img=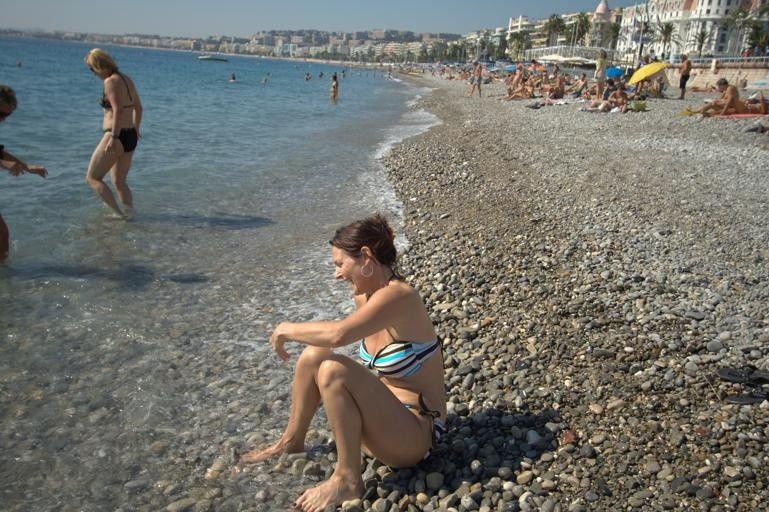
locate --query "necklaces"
[365,274,395,303]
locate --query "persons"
[300,58,406,83]
[228,75,237,82]
[1,83,50,259]
[237,209,450,512]
[329,73,340,104]
[81,48,146,223]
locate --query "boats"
[197,54,227,61]
